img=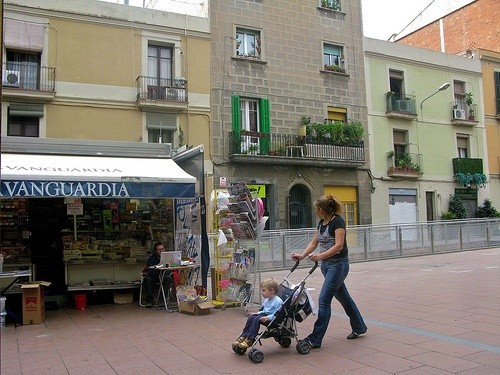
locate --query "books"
[226,180,257,240]
[232,246,255,269]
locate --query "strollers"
[232,255,319,364]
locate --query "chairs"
[138,272,174,307]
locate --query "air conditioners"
[2,70,20,87]
[166,88,185,102]
[453,109,465,119]
[395,100,415,111]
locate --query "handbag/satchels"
[289,292,312,322]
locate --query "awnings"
[1,153,196,198]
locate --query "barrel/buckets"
[73,293,86,310]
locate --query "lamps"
[421,83,451,110]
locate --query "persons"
[291,195,367,350]
[143,242,171,308]
[232,279,283,350]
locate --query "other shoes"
[159,301,165,307]
[146,303,152,308]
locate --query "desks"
[286,146,303,157]
[148,265,200,312]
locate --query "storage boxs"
[15,281,52,325]
[0,297,7,328]
[179,301,214,316]
[211,268,221,300]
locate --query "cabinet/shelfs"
[60,197,175,291]
[0,198,36,293]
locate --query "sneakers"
[347,327,367,339]
[239,338,252,349]
[299,338,321,347]
[232,336,245,346]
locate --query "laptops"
[158,251,182,266]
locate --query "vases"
[270,151,279,156]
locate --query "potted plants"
[466,92,475,120]
[388,151,416,172]
